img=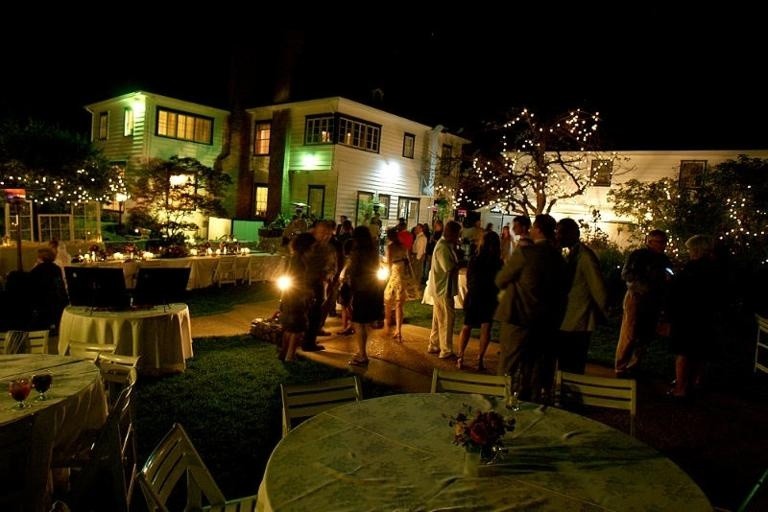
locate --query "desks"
[253,392,714,512]
[422,266,471,310]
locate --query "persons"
[24,248,69,317]
[45,238,61,258]
[273,209,715,402]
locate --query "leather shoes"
[317,330,331,336]
[303,346,325,351]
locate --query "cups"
[506,372,523,412]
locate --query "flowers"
[440,401,517,463]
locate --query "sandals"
[348,359,368,366]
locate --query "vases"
[461,444,482,478]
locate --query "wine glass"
[33,371,53,402]
[10,379,32,412]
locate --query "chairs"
[753,312,768,375]
[430,367,514,400]
[279,374,363,440]
[550,368,637,439]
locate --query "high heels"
[456,356,464,368]
[476,356,483,370]
[394,332,401,342]
